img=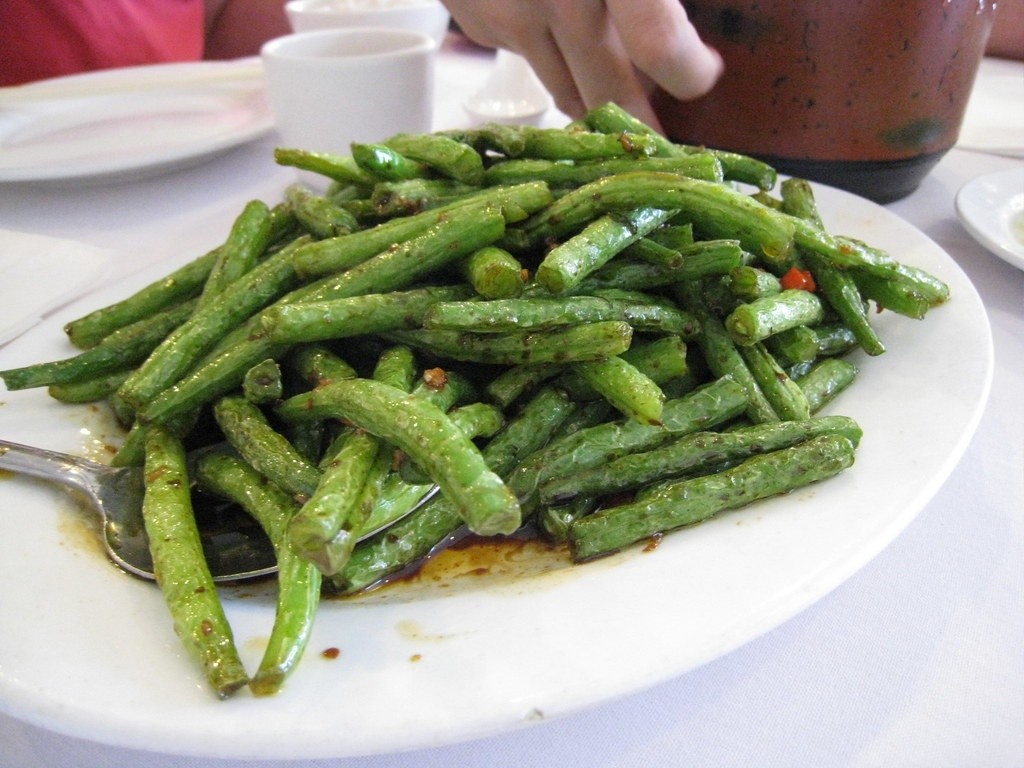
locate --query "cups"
[278,1,453,50]
[261,29,438,193]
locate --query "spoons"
[0,438,444,583]
[468,37,550,127]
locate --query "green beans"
[0,101,950,696]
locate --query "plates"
[953,165,1024,270]
[0,59,283,184]
[0,170,995,764]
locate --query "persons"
[0,0,297,89]
[435,0,725,139]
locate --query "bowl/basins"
[647,0,998,205]
